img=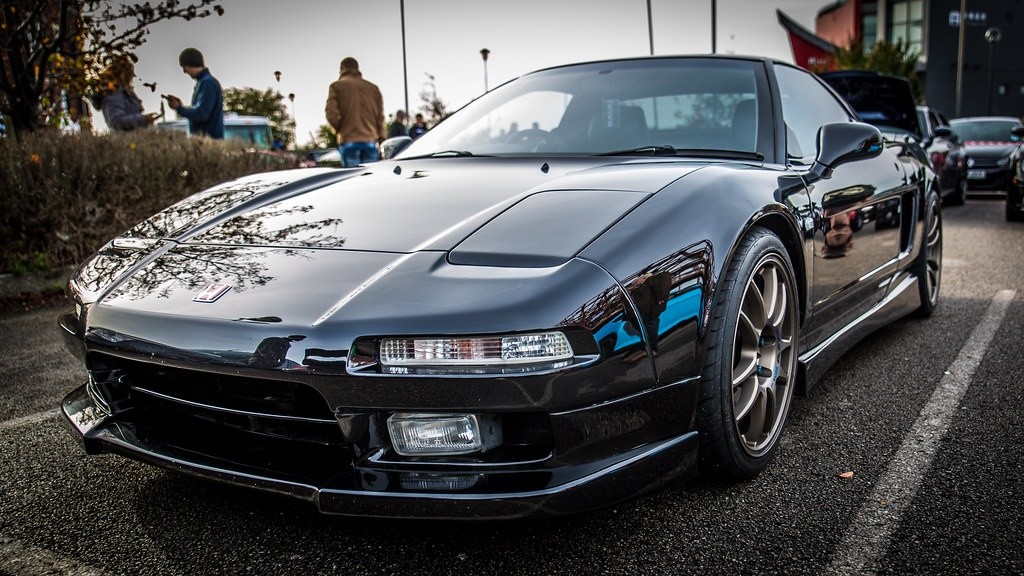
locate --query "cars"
[57,52,942,523]
[820,71,1024,224]
[302,147,345,168]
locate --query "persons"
[325,57,383,168]
[167,47,224,139]
[388,110,407,138]
[409,113,427,140]
[89,61,156,132]
[821,212,855,252]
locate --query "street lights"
[274,69,298,150]
[480,46,492,95]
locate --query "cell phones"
[161,94,180,102]
[153,110,165,119]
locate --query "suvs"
[156,113,286,153]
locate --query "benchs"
[611,105,730,150]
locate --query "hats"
[179,48,203,67]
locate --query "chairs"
[731,99,757,152]
